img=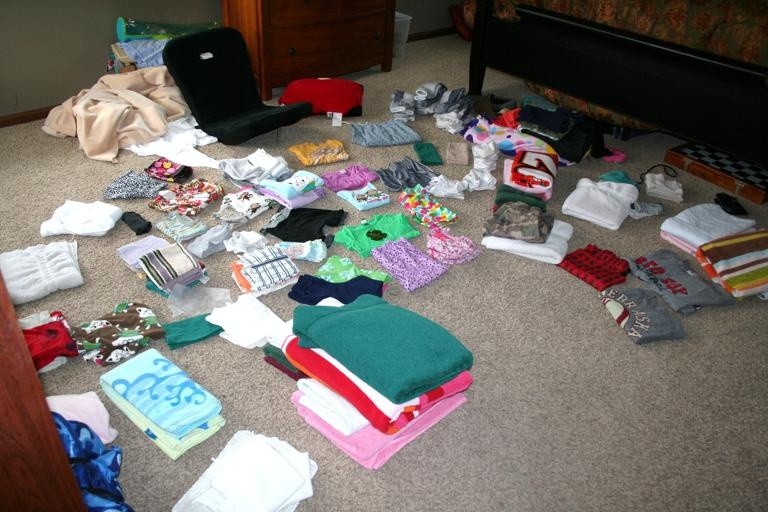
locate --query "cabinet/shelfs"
[223,1,397,101]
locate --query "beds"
[463,0,767,176]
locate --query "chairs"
[162,29,314,146]
